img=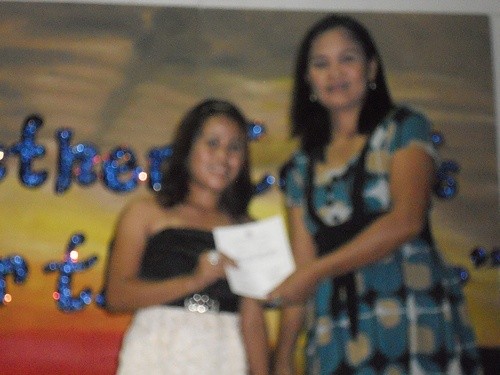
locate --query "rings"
[207,250,219,265]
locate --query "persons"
[267,12,488,375]
[102,95,272,375]
